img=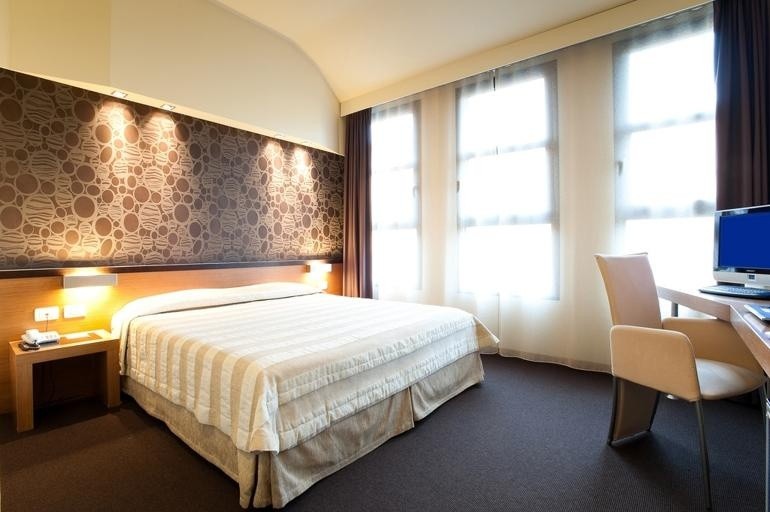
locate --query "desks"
[7,329,121,433]
[650,278,770,374]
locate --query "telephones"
[18,328,60,351]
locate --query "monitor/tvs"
[713,205,770,290]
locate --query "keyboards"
[699,284,770,299]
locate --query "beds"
[111,282,486,509]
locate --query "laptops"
[743,303,770,320]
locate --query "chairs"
[595,253,770,511]
